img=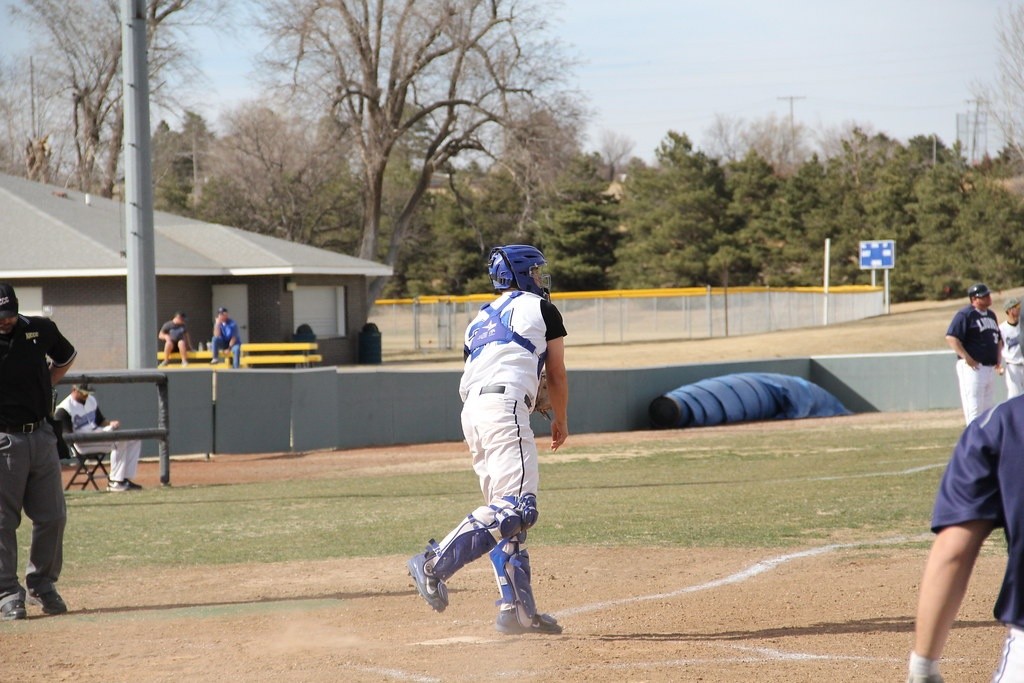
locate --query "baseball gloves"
[533,369,553,423]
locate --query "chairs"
[64,439,110,490]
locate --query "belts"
[0,416,47,435]
[480,386,532,408]
[981,360,998,367]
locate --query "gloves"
[906,652,942,683]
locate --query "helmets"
[488,243,551,299]
[967,284,990,298]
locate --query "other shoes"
[211,358,218,364]
[159,361,167,366]
[181,360,188,366]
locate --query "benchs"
[151,342,323,372]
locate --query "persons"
[945,284,1024,427]
[0,282,76,620]
[408,244,568,635]
[158,311,192,366]
[996,298,1024,399]
[907,296,1024,683]
[55,375,143,492]
[210,307,241,369]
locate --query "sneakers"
[2,600,26,619]
[404,552,445,612]
[497,608,561,635]
[26,584,67,613]
[107,478,142,491]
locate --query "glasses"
[1015,302,1021,309]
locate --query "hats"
[217,307,228,314]
[73,381,97,395]
[0,283,19,318]
[1004,296,1022,312]
